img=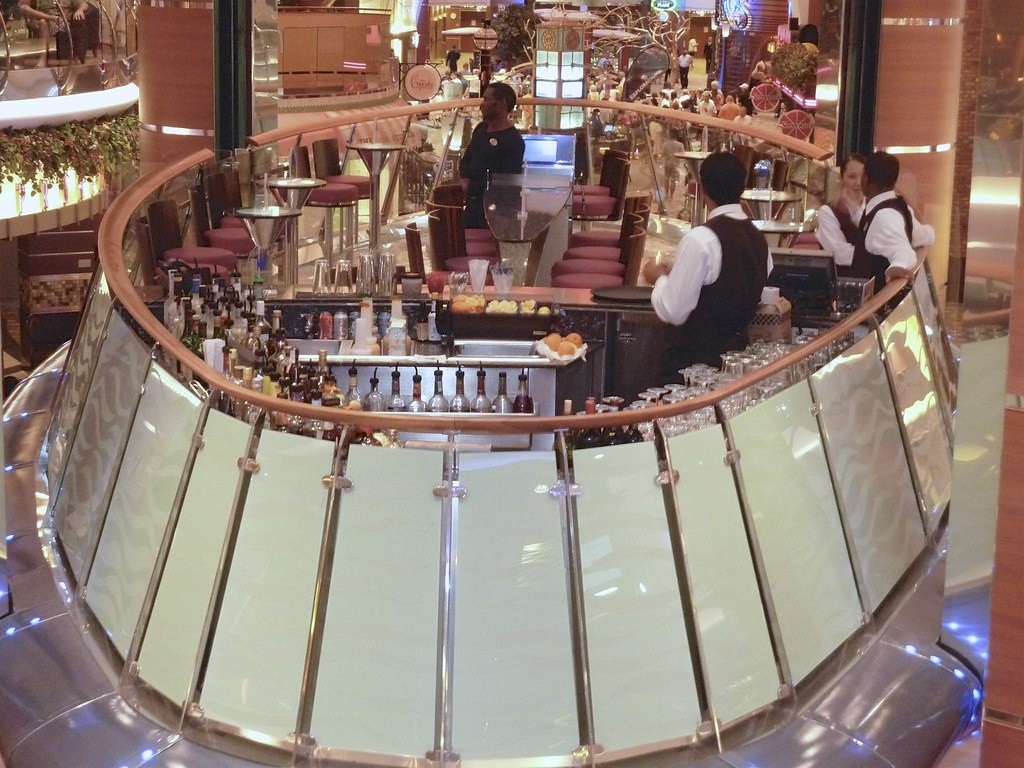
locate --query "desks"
[256,178,328,283]
[484,135,576,285]
[346,143,404,256]
[739,190,801,225]
[752,219,818,247]
[234,207,304,290]
[674,152,711,228]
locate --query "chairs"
[134,136,790,293]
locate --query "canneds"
[320,310,360,340]
[373,311,391,338]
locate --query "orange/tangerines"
[545,332,582,357]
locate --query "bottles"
[163,257,533,446]
[556,396,644,470]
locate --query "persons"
[587,33,772,147]
[458,82,526,256]
[815,150,936,309]
[442,63,532,95]
[446,45,461,74]
[18,0,88,60]
[679,49,694,89]
[642,151,774,385]
[662,129,684,200]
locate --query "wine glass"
[630,330,854,442]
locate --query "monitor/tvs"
[767,247,841,310]
[520,133,577,181]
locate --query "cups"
[377,254,395,297]
[356,255,374,298]
[489,264,514,292]
[334,259,353,296]
[468,259,489,292]
[312,259,332,297]
